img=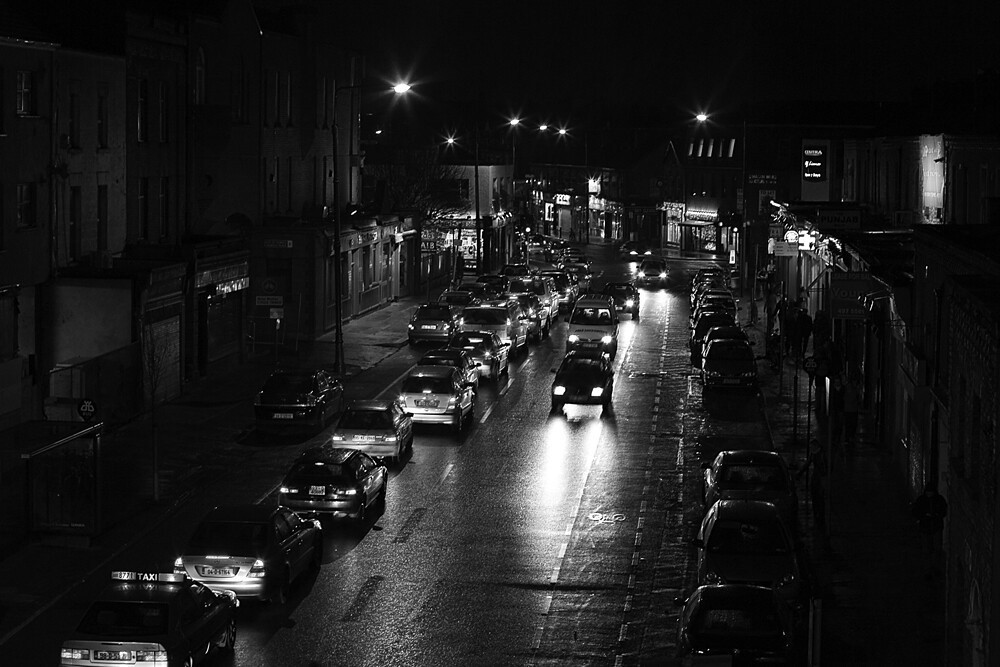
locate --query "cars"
[598,281,640,318]
[416,229,593,395]
[280,447,388,525]
[548,349,615,411]
[673,584,801,666]
[699,448,799,528]
[635,255,671,288]
[408,303,456,346]
[683,256,775,450]
[686,498,804,601]
[60,570,240,667]
[620,242,650,261]
[330,400,414,464]
[172,501,324,609]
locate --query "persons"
[762,286,864,449]
[793,438,828,532]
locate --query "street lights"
[319,206,358,369]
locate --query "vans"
[563,293,619,360]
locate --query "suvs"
[397,366,474,434]
[251,369,344,438]
